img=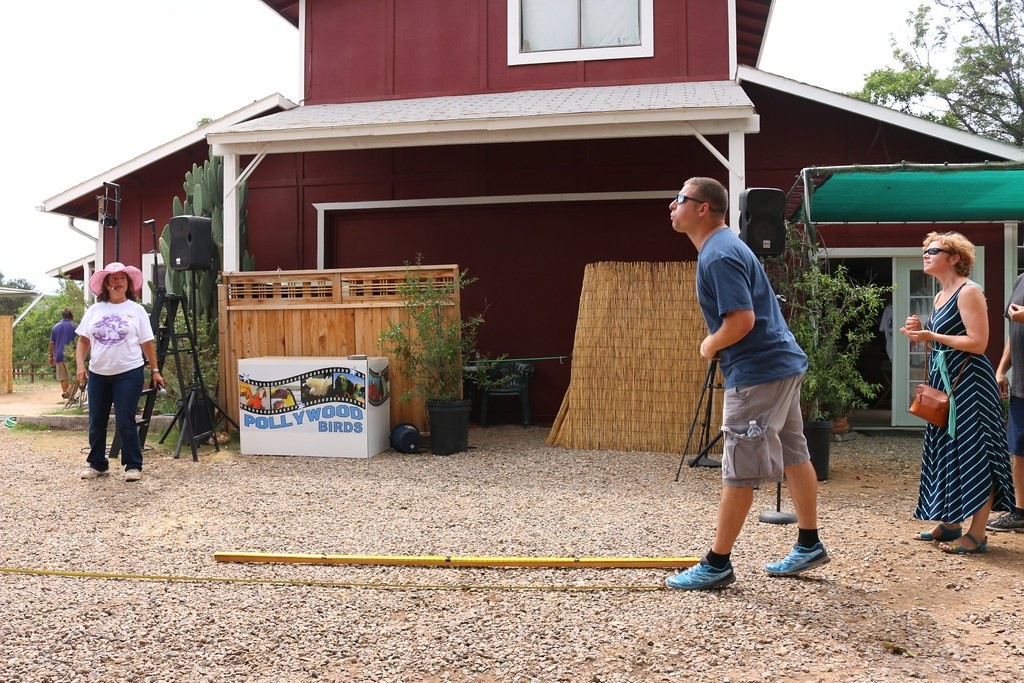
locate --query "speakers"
[738,187,787,258]
[166,215,212,272]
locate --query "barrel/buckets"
[389,423,421,453]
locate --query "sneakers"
[762,539,832,578]
[665,557,736,590]
[125,468,142,481]
[81,468,110,479]
[986,510,1024,533]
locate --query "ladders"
[108,286,221,462]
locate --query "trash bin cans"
[390,423,422,452]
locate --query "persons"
[665,176,831,591]
[48,306,79,404]
[985,270,1024,533]
[75,262,165,482]
[900,231,1016,552]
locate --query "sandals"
[912,525,961,540]
[938,533,988,554]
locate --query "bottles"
[748,420,760,437]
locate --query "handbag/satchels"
[908,383,951,430]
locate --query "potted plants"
[759,217,897,482]
[375,252,520,455]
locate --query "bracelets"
[151,368,160,373]
[49,355,53,357]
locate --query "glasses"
[924,248,952,257]
[675,194,714,214]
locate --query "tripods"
[159,270,240,459]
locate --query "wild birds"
[302,376,332,398]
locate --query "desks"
[237,355,392,460]
[460,364,483,425]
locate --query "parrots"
[335,375,349,398]
[240,386,266,410]
[351,383,362,400]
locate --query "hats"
[89,262,143,298]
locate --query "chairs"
[868,339,892,407]
[482,363,537,428]
